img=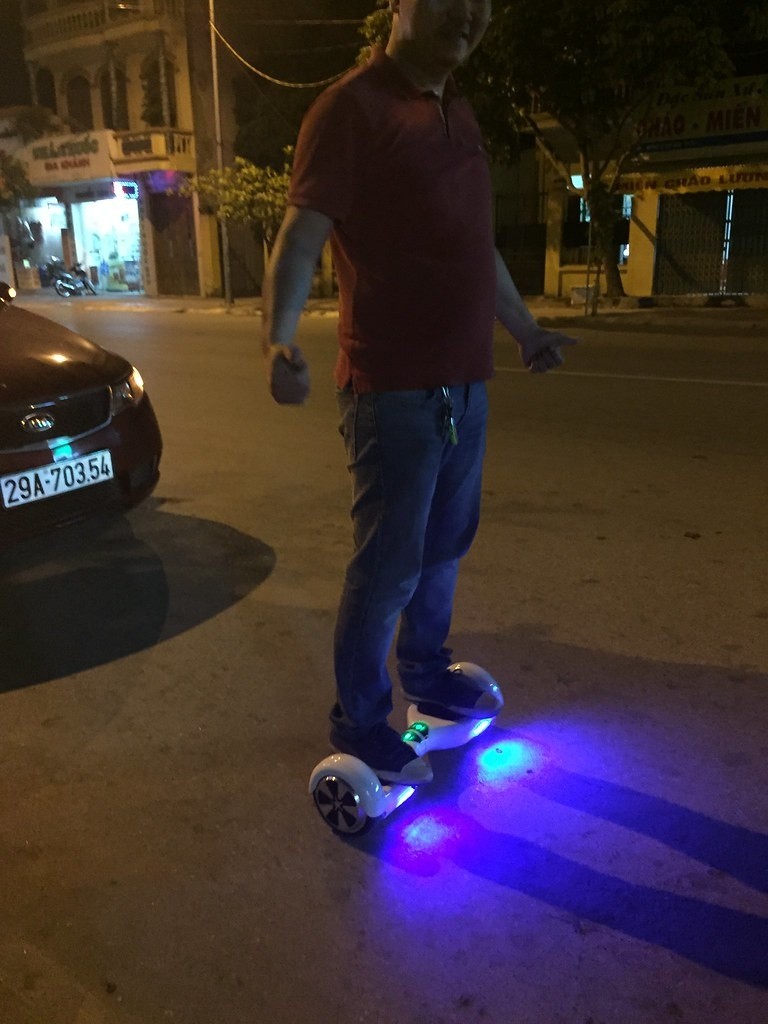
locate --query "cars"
[0,280,164,553]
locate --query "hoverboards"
[307,663,499,837]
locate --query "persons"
[262,0,578,786]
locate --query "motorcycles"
[54,262,100,299]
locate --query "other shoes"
[327,727,434,786]
[400,667,502,718]
